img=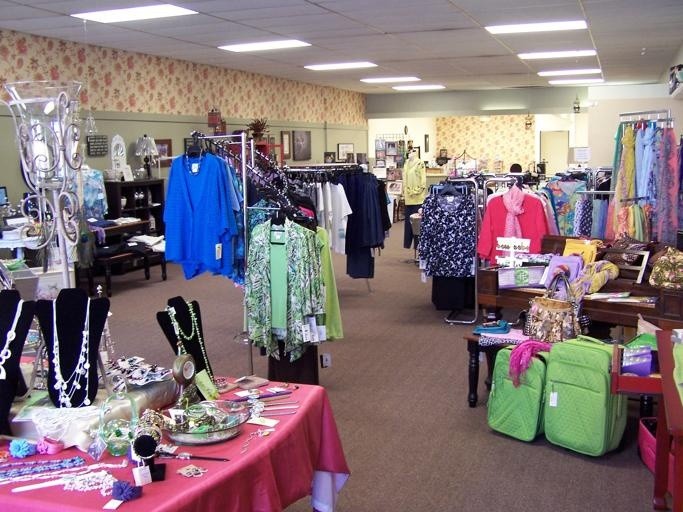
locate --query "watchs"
[231,390,301,418]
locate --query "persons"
[402,153,426,249]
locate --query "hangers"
[428,173,544,199]
[191,126,364,229]
[617,109,674,132]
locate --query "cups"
[120,198,127,209]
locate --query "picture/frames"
[153,138,173,168]
[373,128,427,203]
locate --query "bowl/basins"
[162,399,252,444]
[134,170,147,179]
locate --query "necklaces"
[165,304,211,380]
[51,297,93,409]
[3,446,127,497]
[0,299,27,385]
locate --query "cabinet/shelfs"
[100,175,168,281]
[463,234,683,424]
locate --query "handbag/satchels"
[602,235,651,271]
[524,296,582,343]
[649,246,683,294]
[497,238,597,290]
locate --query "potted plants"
[244,118,267,142]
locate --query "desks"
[0,351,354,511]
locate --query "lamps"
[134,134,157,179]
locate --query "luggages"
[487,335,628,457]
